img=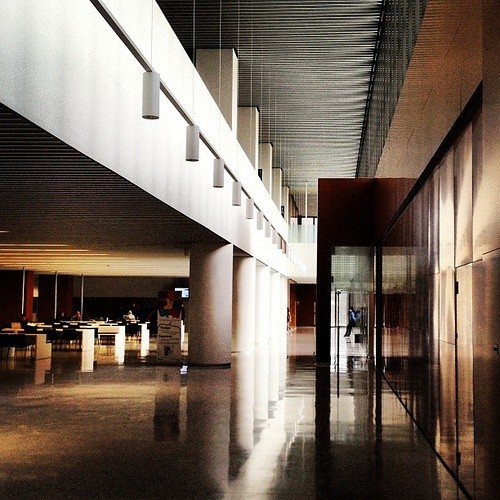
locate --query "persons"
[344,307,356,336]
[125,310,136,321]
[71,311,82,321]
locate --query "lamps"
[139,0,307,277]
[32,272,40,298]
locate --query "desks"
[25,327,95,352]
[98,323,126,347]
[0,331,47,360]
[119,321,151,341]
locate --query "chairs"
[11,321,21,329]
